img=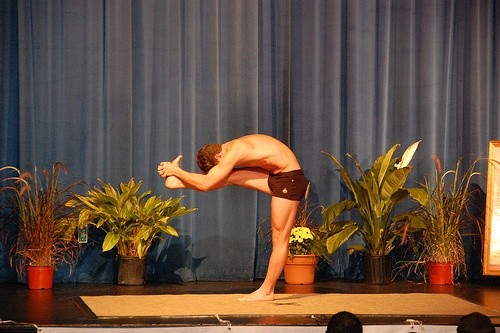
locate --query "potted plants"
[320,139,422,285]
[0,162,89,289]
[56,177,198,286]
[412,153,500,285]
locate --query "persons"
[326,311,363,333]
[456,312,496,333]
[157,134,306,301]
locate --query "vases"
[284,254,320,285]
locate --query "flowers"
[287,225,316,254]
